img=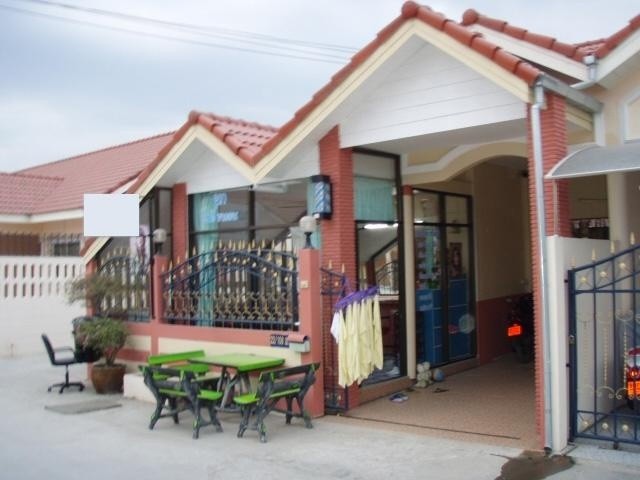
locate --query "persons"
[133,227,148,257]
[446,247,462,279]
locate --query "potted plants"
[65,269,132,394]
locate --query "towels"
[329,291,385,388]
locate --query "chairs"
[40,333,84,394]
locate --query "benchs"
[137,350,321,443]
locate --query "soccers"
[432,368,445,382]
